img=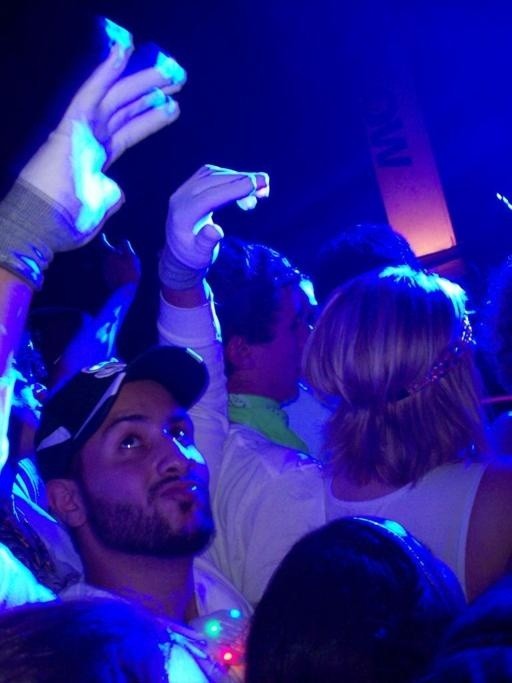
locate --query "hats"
[34,345,209,478]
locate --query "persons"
[0,40,510,683]
